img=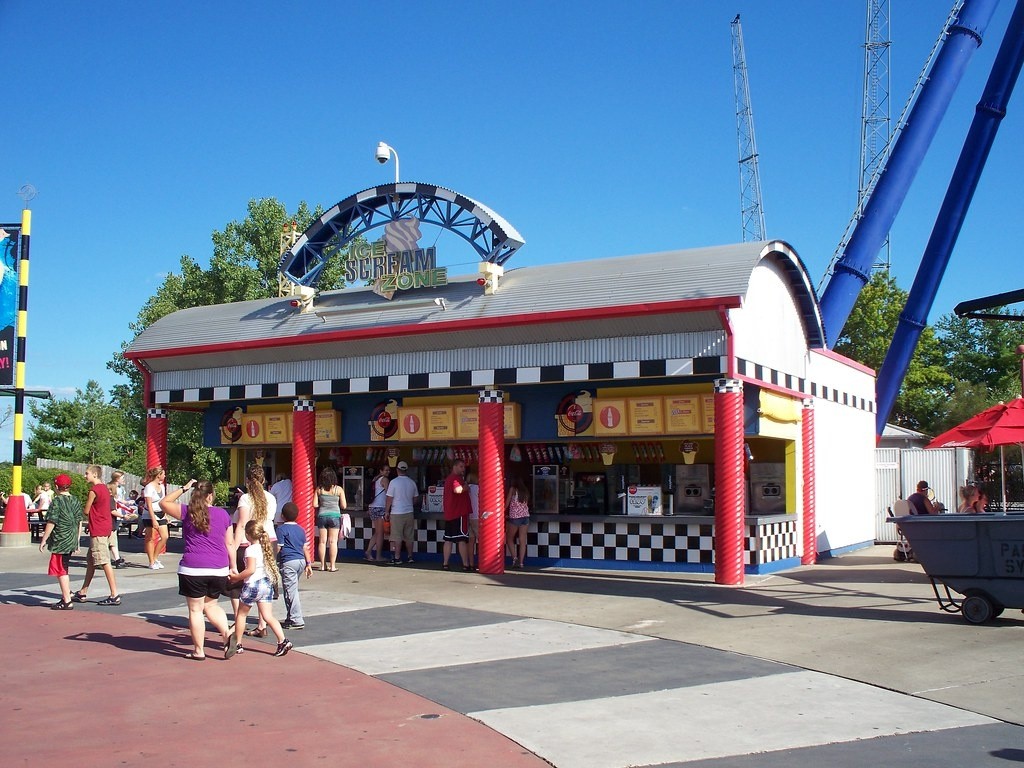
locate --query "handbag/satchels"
[155,511,165,519]
[384,521,390,535]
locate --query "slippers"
[374,556,387,562]
[183,652,205,660]
[363,557,373,561]
[223,633,237,659]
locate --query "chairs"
[894,500,909,517]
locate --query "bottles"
[647,495,658,513]
[251,421,255,436]
[409,415,415,432]
[607,407,613,427]
[524,443,601,464]
[631,441,665,462]
[413,447,478,466]
[366,447,383,465]
[422,495,425,509]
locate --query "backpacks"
[365,475,384,504]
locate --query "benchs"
[119,521,184,539]
[27,520,48,540]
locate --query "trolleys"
[884,510,1024,626]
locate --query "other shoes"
[155,560,165,569]
[148,564,158,570]
[136,535,145,539]
[131,531,138,538]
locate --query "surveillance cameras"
[375,146,390,163]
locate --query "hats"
[917,481,932,491]
[55,474,72,488]
[397,462,408,470]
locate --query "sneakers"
[280,621,304,629]
[274,638,293,656]
[236,644,243,654]
[407,557,415,563]
[386,556,403,564]
[116,559,132,569]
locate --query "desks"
[25,509,49,531]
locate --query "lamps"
[477,278,492,287]
[314,310,327,323]
[434,297,446,311]
[283,221,297,233]
[290,295,316,309]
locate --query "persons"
[441,458,478,571]
[365,461,419,564]
[505,476,531,569]
[314,468,347,572]
[908,481,941,515]
[0,482,55,519]
[75,465,122,606]
[39,474,83,610]
[958,485,986,514]
[160,464,313,661]
[96,465,171,569]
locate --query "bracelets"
[306,561,311,565]
[181,486,186,492]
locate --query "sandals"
[98,595,121,605]
[46,596,73,610]
[511,557,518,569]
[470,566,475,571]
[517,564,524,568]
[442,563,448,570]
[462,566,469,572]
[71,591,87,604]
[246,627,268,638]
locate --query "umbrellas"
[923,395,1024,516]
